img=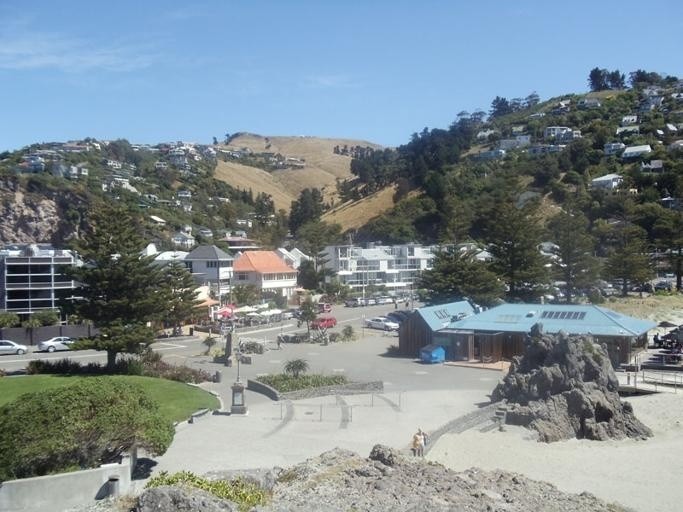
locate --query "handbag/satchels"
[424,435,430,445]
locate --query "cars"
[315,303,332,313]
[345,294,420,307]
[311,316,337,329]
[38,336,74,353]
[523,278,673,301]
[281,307,302,320]
[365,310,411,331]
[0,340,27,355]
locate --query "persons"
[654,333,662,347]
[410,426,428,457]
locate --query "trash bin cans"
[216,370,222,383]
[108,475,120,497]
[190,328,193,336]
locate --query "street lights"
[234,349,242,386]
